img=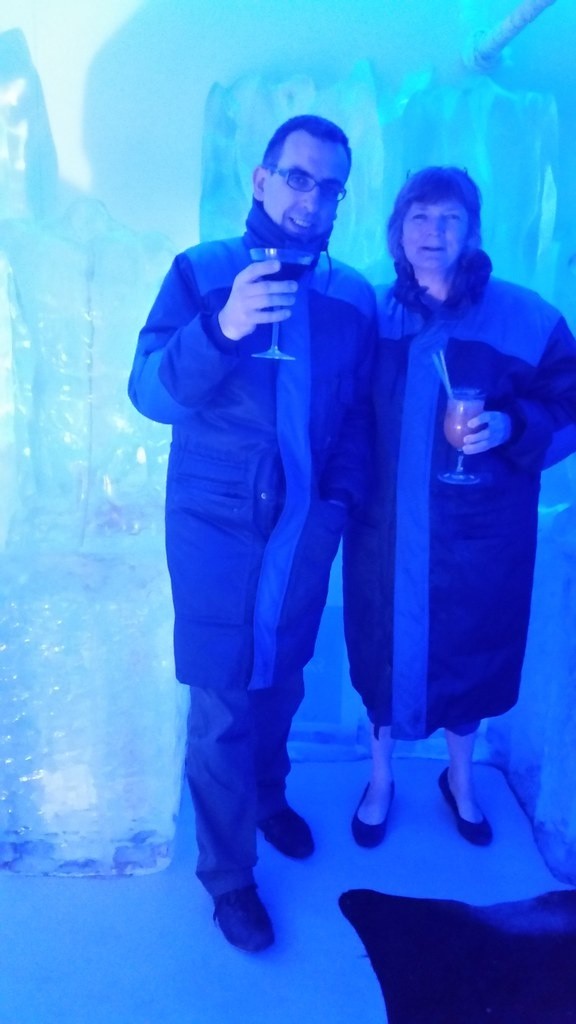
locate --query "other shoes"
[256,804,322,860]
[440,768,493,846]
[351,783,395,849]
[211,883,276,951]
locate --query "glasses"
[271,167,350,203]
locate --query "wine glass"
[250,248,315,360]
[438,388,486,485]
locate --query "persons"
[127,113,381,955]
[346,165,573,846]
